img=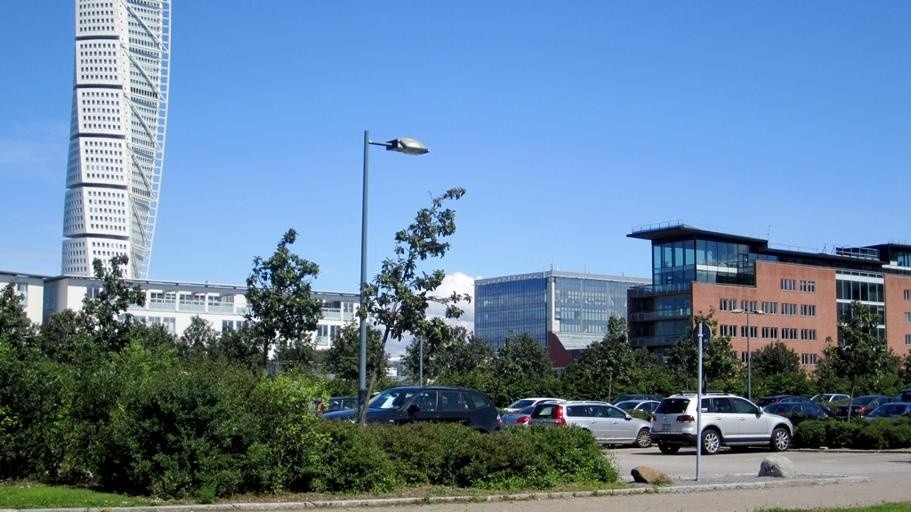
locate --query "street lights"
[356,130,431,428]
[731,307,766,403]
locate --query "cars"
[322,397,359,413]
[324,387,498,435]
